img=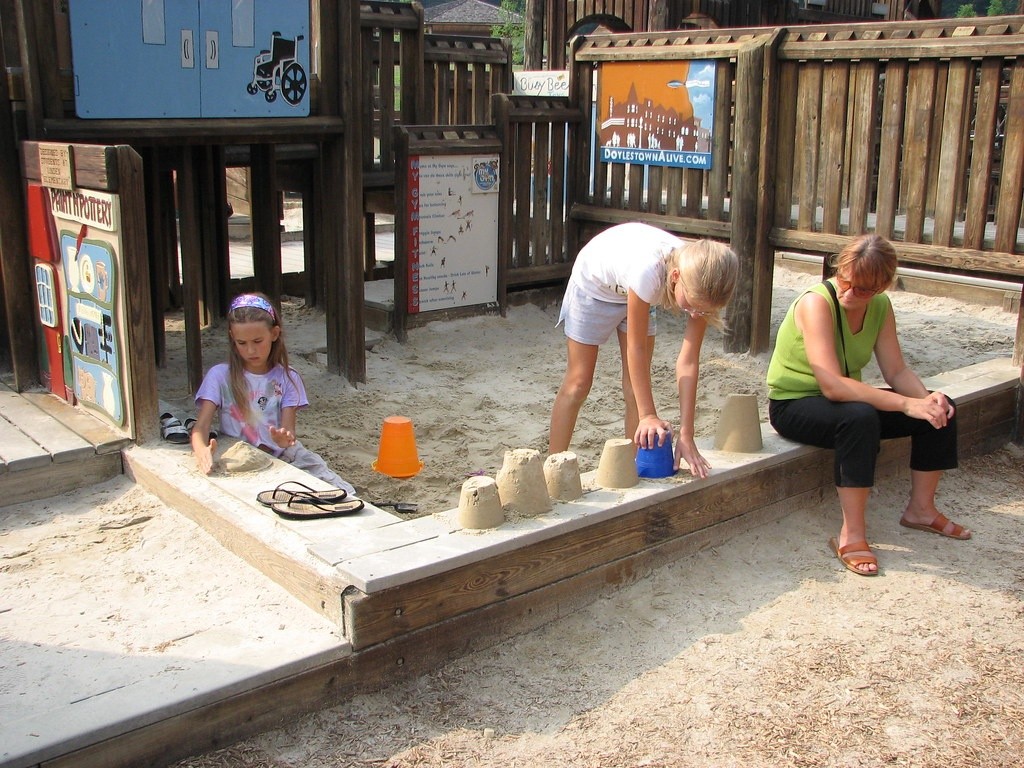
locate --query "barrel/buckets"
[373,416,421,478]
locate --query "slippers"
[828,536,878,576]
[255,480,363,521]
[899,511,971,540]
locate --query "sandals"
[161,412,219,443]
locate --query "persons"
[765,234,971,576]
[190,293,358,498]
[548,223,738,481]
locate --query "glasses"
[837,276,883,299]
[682,284,719,316]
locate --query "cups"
[638,435,675,478]
